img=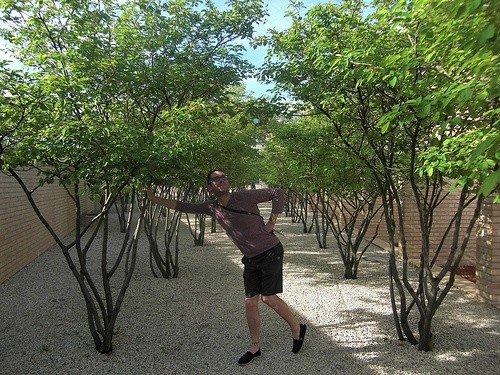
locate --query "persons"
[146,168,307,366]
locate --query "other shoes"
[291,323,307,353]
[239,348,261,365]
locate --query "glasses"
[208,175,229,184]
